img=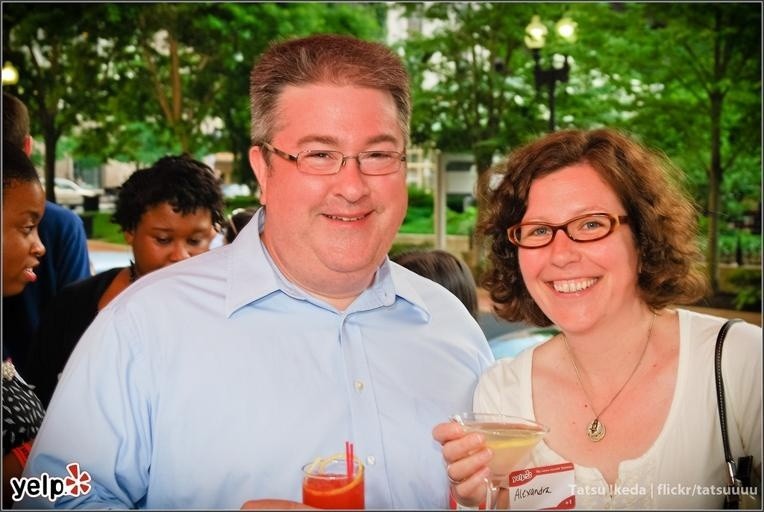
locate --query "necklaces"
[560,309,655,444]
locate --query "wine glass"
[447,410,550,509]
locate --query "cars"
[39,176,107,210]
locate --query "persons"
[16,36,493,510]
[391,250,480,323]
[432,129,763,512]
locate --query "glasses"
[263,141,405,175]
[507,211,630,249]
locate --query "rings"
[445,464,466,485]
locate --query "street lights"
[523,14,577,132]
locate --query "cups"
[303,456,366,511]
